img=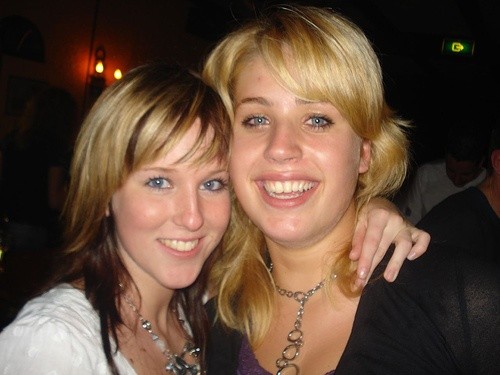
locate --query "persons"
[0,62,431,375]
[0,14,71,321]
[199,3,500,375]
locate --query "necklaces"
[117,281,200,375]
[264,257,339,375]
